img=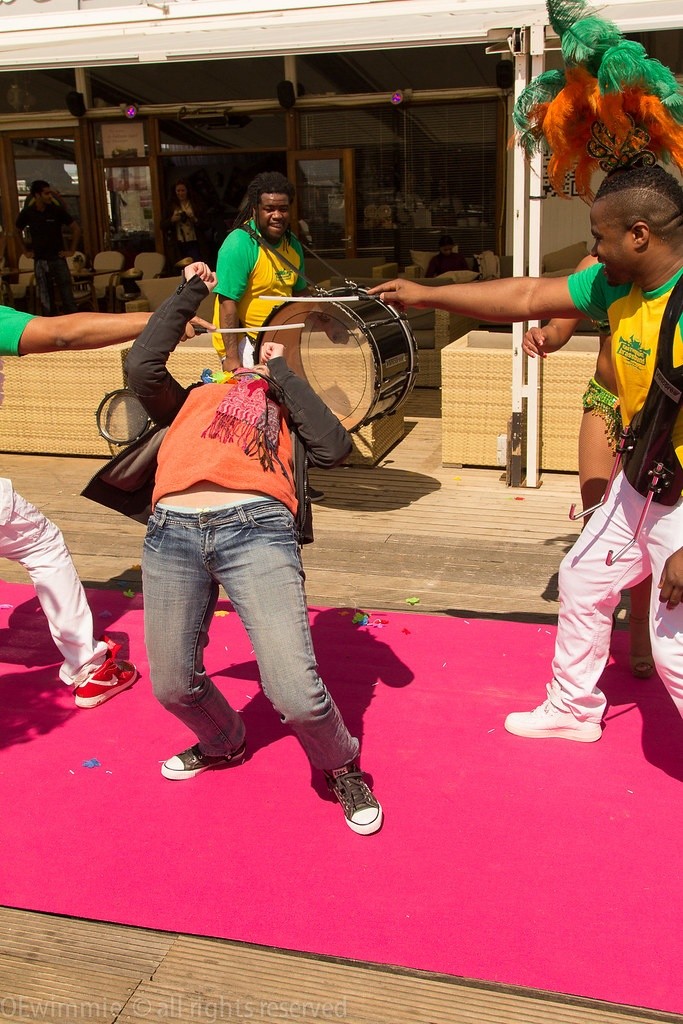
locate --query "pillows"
[436,270,480,283]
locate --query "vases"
[73,261,84,271]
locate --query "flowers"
[73,254,83,262]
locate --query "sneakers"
[75,635,138,708]
[161,740,246,780]
[322,762,383,835]
[505,683,602,743]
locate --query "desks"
[68,268,122,312]
[1,266,34,308]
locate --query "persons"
[0,304,217,709]
[523,254,655,679]
[368,166,683,742]
[211,172,326,501]
[164,179,202,268]
[424,235,470,278]
[80,262,381,835]
[15,180,79,317]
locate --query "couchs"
[125,249,513,387]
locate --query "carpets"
[0,580,683,1015]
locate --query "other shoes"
[306,487,323,503]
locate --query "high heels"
[630,615,655,677]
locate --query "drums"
[251,287,420,434]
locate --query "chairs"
[3,252,165,313]
